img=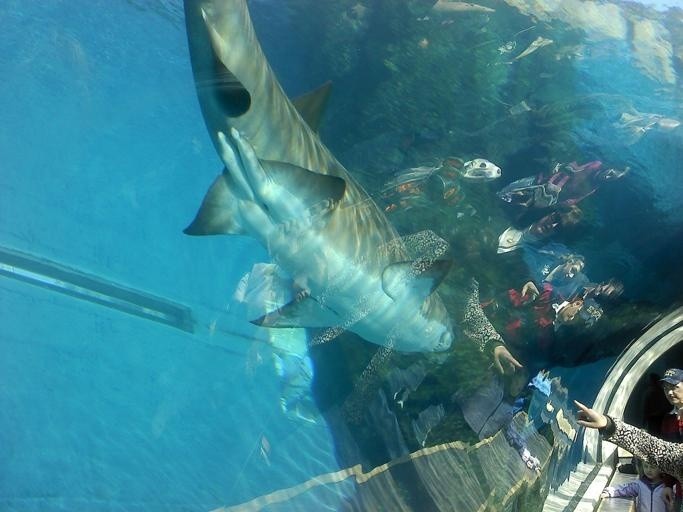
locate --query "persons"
[306,160,634,477]
[573,367,683,511]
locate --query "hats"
[660,369,683,385]
